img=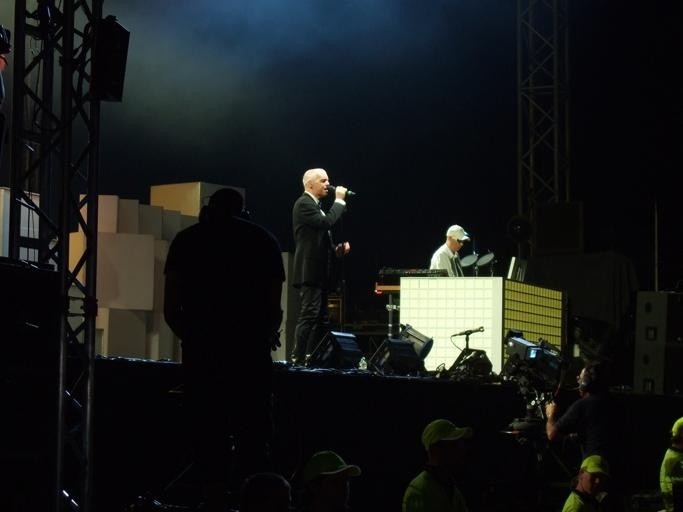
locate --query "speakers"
[367,339,417,376]
[448,348,491,374]
[633,290,683,395]
[307,330,362,370]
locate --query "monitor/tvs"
[507,257,528,282]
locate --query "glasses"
[453,237,463,243]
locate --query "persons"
[162,187,286,364]
[544,364,614,470]
[287,166,351,370]
[235,472,294,511]
[560,454,610,512]
[401,418,474,512]
[429,224,472,277]
[658,416,682,512]
[298,450,363,510]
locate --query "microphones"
[327,185,356,195]
[458,327,484,335]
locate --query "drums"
[461,254,478,267]
[476,253,495,267]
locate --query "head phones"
[581,365,591,391]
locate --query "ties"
[454,258,463,277]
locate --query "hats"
[671,415,683,438]
[302,450,362,478]
[421,418,472,450]
[446,224,470,241]
[579,453,610,476]
